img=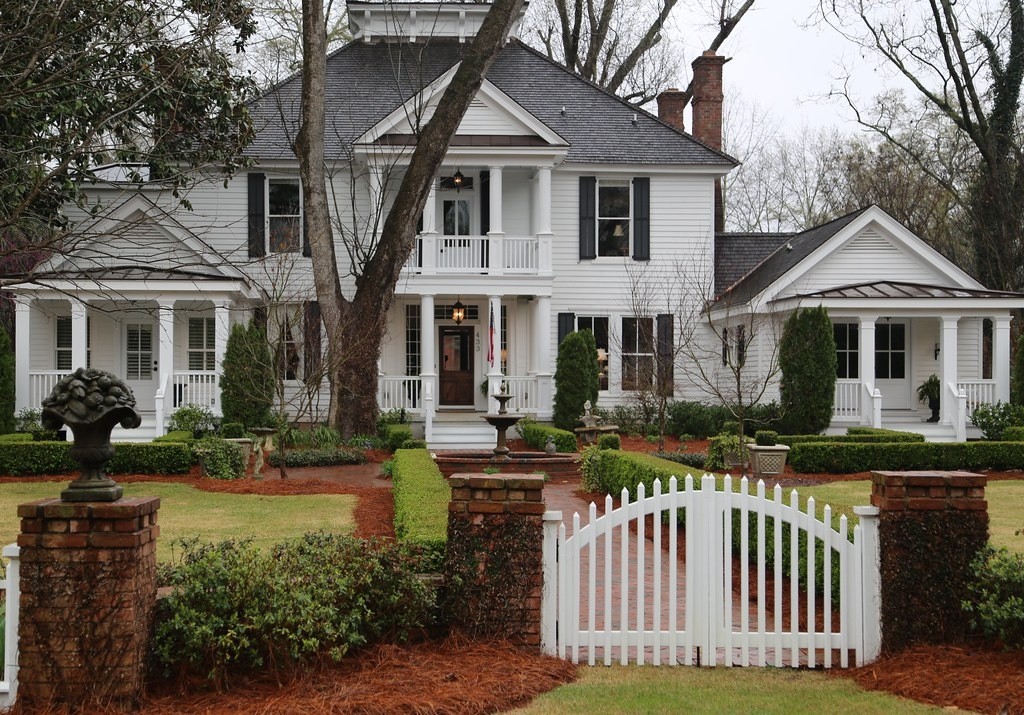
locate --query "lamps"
[452,294,464,327]
[613,225,625,249]
[933,335,940,360]
[596,347,607,376]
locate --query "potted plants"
[713,421,752,466]
[917,373,941,423]
[746,429,790,476]
[453,165,463,194]
[217,423,251,468]
